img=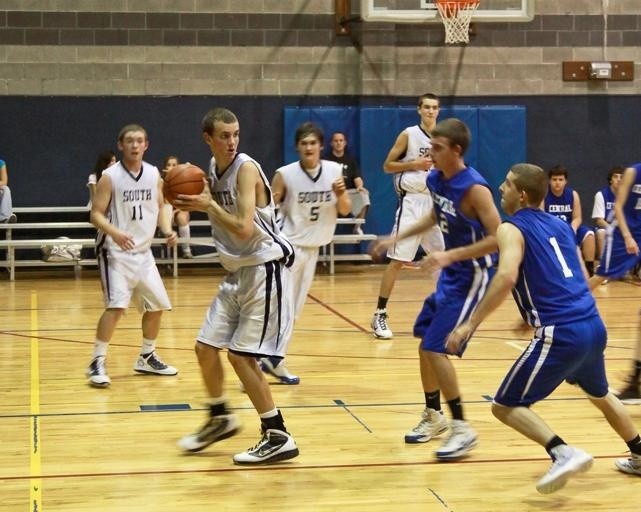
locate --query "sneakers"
[614,450,641,475]
[371,308,394,340]
[234,410,298,465]
[613,361,641,405]
[5,215,17,224]
[177,403,243,452]
[434,420,480,460]
[536,445,594,494]
[85,356,111,387]
[256,356,300,385]
[405,407,448,443]
[134,350,178,376]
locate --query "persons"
[367,94,449,343]
[87,125,179,390]
[162,156,193,257]
[86,150,118,211]
[327,130,372,238]
[539,167,594,284]
[171,105,300,466]
[582,158,641,292]
[613,300,641,401]
[442,162,641,498]
[237,124,352,397]
[368,116,501,462]
[587,169,625,263]
[0,158,17,226]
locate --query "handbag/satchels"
[41,236,83,262]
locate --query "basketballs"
[162,164,206,209]
